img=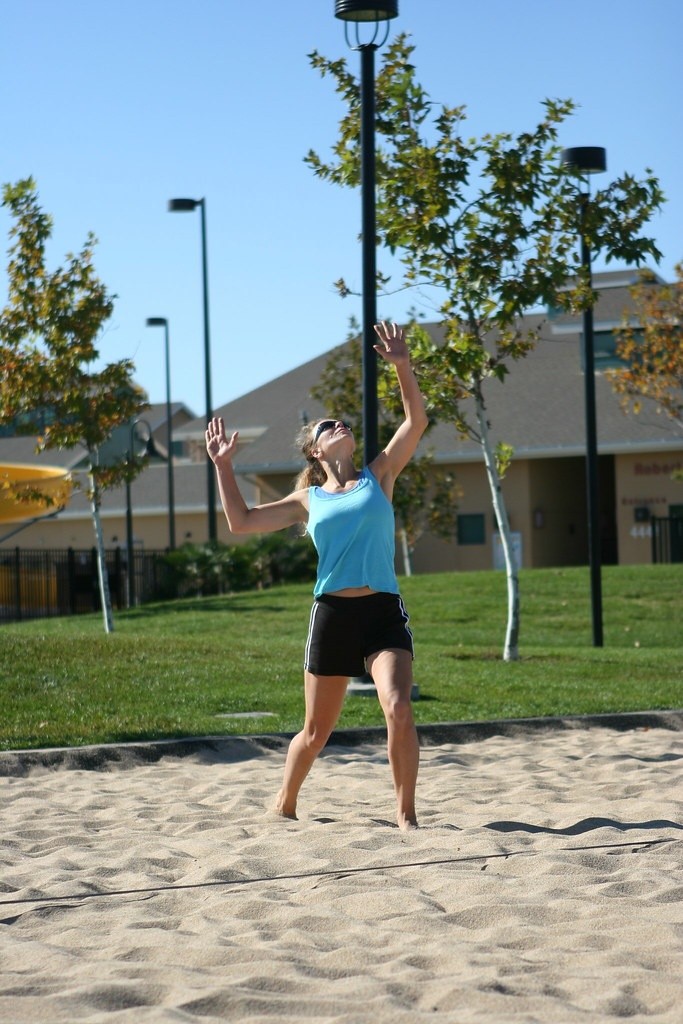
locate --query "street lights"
[556,144,609,646]
[327,0,406,689]
[124,417,162,619]
[146,316,181,602]
[168,194,221,595]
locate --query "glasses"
[314,420,353,442]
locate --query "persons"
[205,321,430,831]
